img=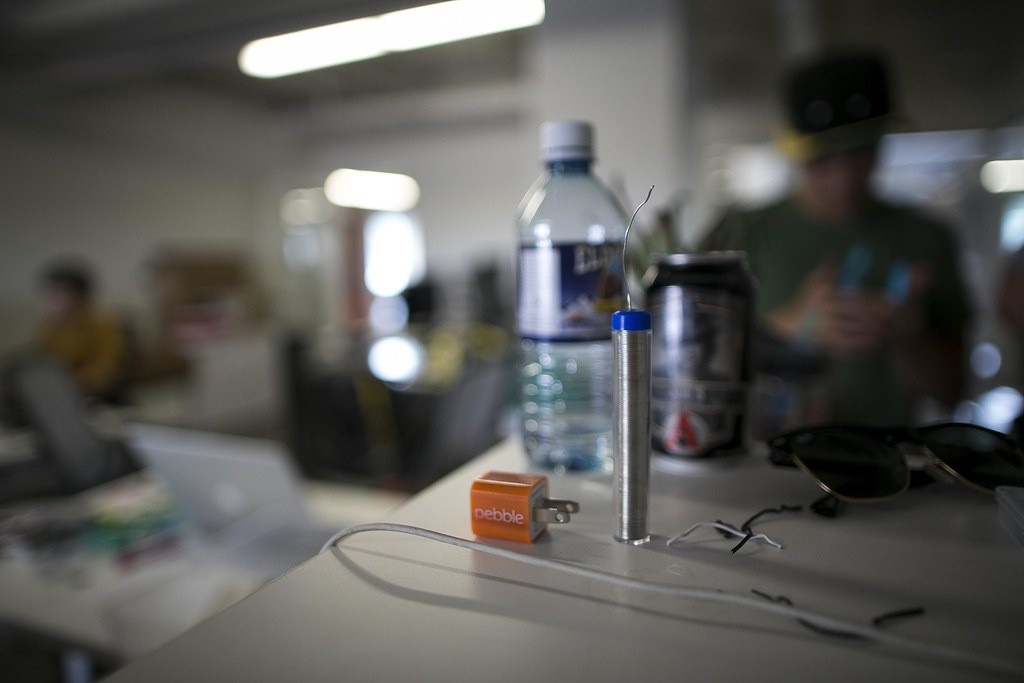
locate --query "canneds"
[639,251,758,474]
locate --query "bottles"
[509,120,631,473]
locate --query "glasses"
[766,423,1024,516]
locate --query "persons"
[19,261,143,412]
[699,42,971,460]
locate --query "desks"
[0,434,410,682]
[97,436,1023,682]
